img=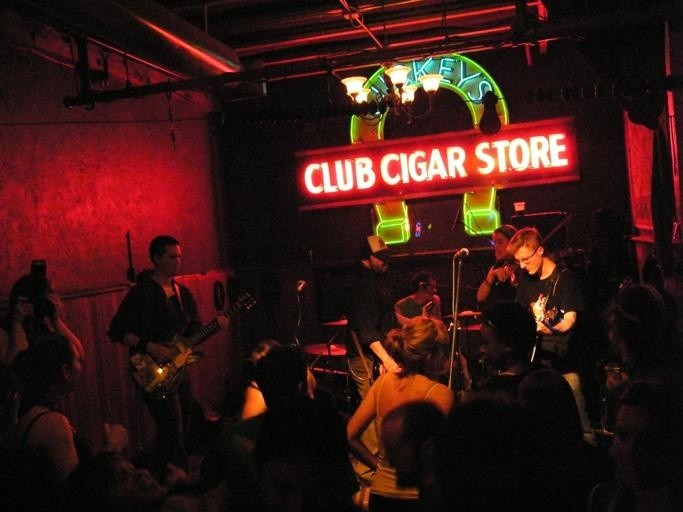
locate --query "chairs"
[446,308,484,358]
[306,317,350,394]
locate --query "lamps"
[340,1,444,127]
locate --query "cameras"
[24,260,53,317]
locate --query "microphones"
[419,282,431,288]
[454,247,469,259]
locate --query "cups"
[604,361,622,382]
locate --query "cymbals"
[440,310,481,319]
[304,343,347,357]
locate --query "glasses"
[515,249,537,265]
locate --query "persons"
[345,233,400,345]
[237,313,317,421]
[103,235,214,438]
[345,316,455,512]
[446,225,683,511]
[377,400,449,511]
[1,270,210,512]
[392,270,443,323]
[232,343,353,512]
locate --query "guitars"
[130,294,255,399]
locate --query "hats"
[360,235,392,260]
